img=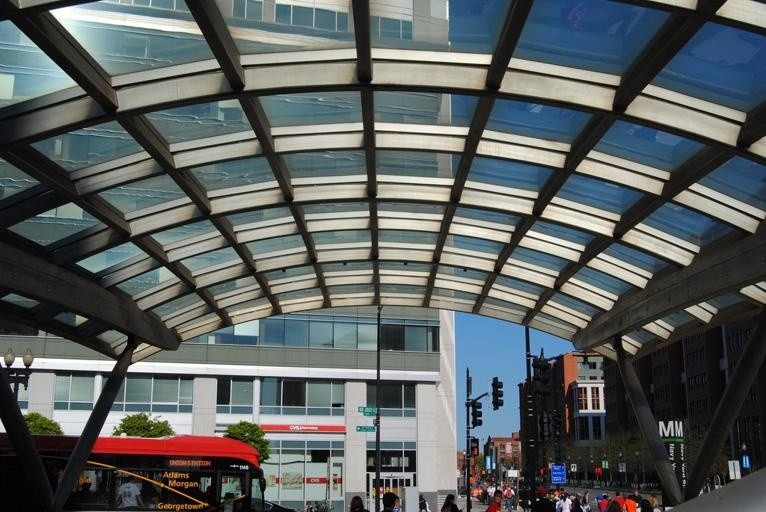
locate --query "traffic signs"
[550,463,567,484]
[357,406,377,432]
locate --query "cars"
[221,495,294,512]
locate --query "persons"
[382,492,400,511]
[440,494,459,511]
[419,495,427,510]
[306,500,324,511]
[350,496,366,510]
[65,471,211,512]
[520,484,590,511]
[596,487,662,511]
[470,473,516,511]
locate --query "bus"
[0,434,265,511]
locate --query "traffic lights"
[493,377,503,410]
[470,438,479,458]
[471,402,482,426]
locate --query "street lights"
[569,452,641,489]
